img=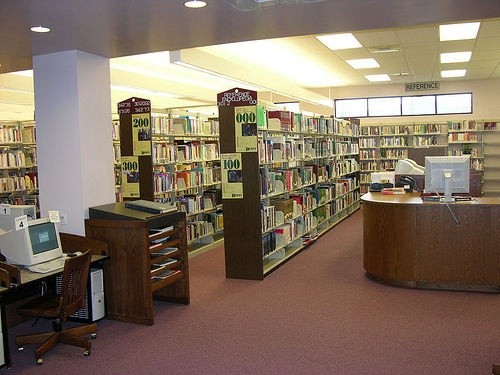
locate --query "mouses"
[73,251,83,256]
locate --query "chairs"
[15,248,99,364]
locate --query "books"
[0,110,497,279]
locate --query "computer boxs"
[39,268,105,323]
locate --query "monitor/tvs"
[0,217,63,266]
[395,160,425,175]
[425,156,471,202]
[406,159,426,170]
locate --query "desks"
[0,232,109,369]
[362,190,500,293]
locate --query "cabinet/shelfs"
[0,88,500,326]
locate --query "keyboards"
[30,257,70,273]
